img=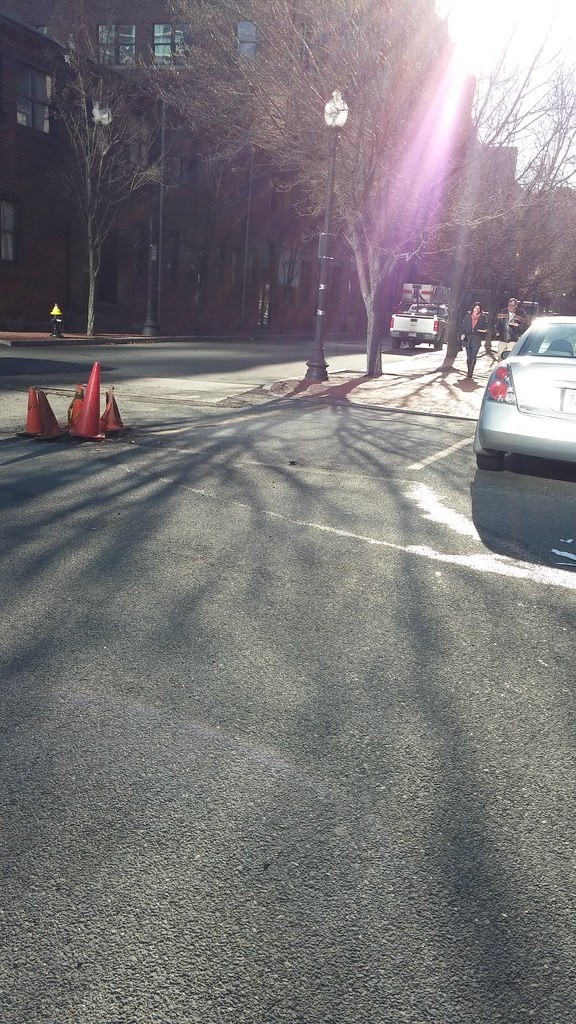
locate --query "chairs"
[547,339,574,356]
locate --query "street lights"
[305,85,350,380]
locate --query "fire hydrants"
[50,302,64,338]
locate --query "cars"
[462,301,562,337]
[473,315,576,472]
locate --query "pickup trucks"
[390,304,450,351]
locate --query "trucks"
[399,282,451,311]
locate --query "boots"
[467,359,475,378]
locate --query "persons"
[496,298,526,364]
[462,302,487,379]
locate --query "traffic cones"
[25,361,124,441]
